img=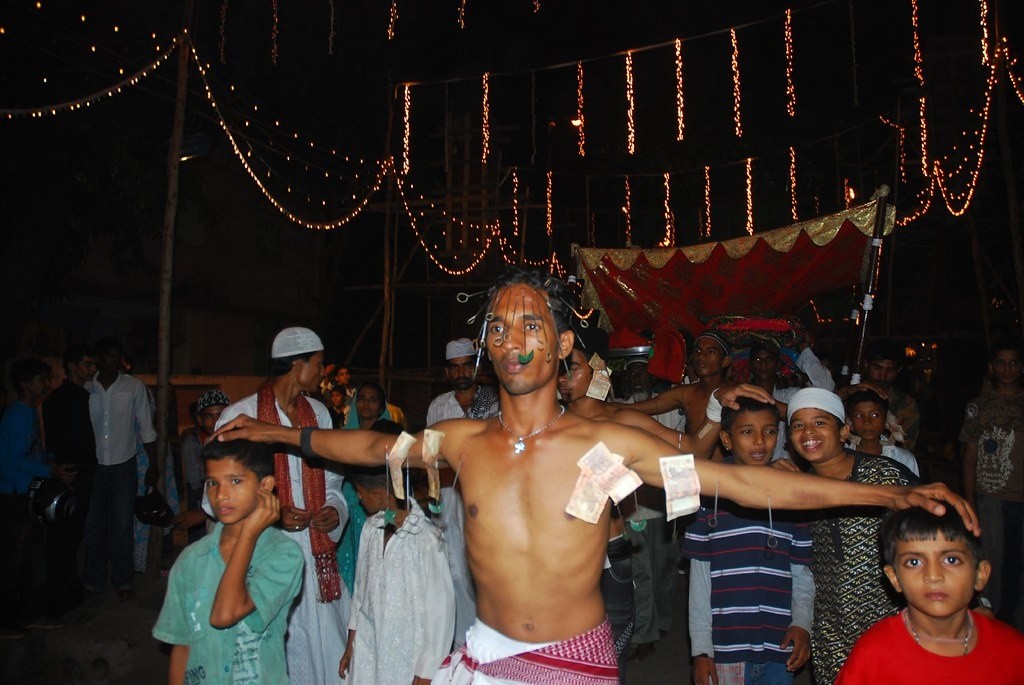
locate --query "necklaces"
[498,404,565,454]
[905,610,970,655]
[902,607,974,643]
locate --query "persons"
[0,266,1024,685]
[201,266,982,685]
[152,437,306,685]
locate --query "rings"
[295,526,299,530]
[294,515,298,520]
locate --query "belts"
[607,547,632,562]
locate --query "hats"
[196,389,231,413]
[623,357,648,370]
[271,326,325,361]
[694,329,733,356]
[786,387,845,425]
[446,338,478,360]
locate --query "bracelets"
[707,387,722,423]
[678,431,682,450]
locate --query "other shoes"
[0,622,27,639]
[85,579,105,593]
[628,642,654,660]
[120,588,138,602]
[25,615,66,629]
